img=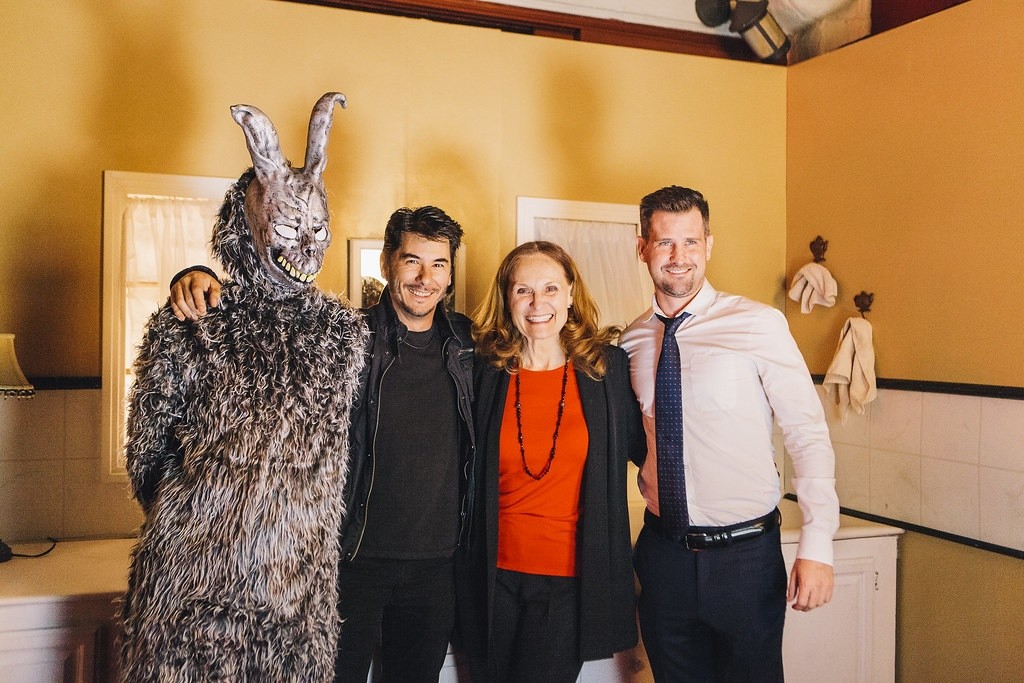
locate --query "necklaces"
[403,321,437,349]
[516,355,568,480]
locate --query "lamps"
[0,333,37,563]
[695,0,791,64]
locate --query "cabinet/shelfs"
[0,603,126,682]
[429,537,896,682]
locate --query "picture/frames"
[349,238,467,315]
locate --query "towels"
[822,316,877,429]
[788,262,838,314]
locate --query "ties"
[654,312,693,543]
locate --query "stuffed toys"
[111,169,369,683]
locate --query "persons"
[615,186,840,683]
[168,206,484,683]
[449,241,647,683]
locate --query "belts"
[644,507,779,550]
